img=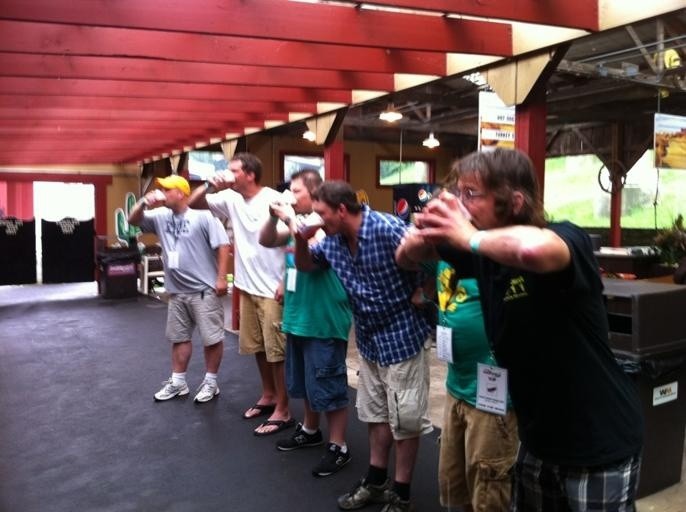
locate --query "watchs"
[268,212,279,223]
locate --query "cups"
[408,191,473,243]
[293,210,324,234]
[276,188,297,207]
[203,170,233,190]
[142,187,167,207]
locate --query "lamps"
[377,100,402,124]
[300,130,314,143]
[419,131,441,151]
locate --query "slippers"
[243,402,276,419]
[253,415,295,437]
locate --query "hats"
[156,174,190,197]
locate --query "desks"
[596,249,662,282]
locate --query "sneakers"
[311,442,352,477]
[193,382,220,403]
[337,476,389,510]
[154,378,190,401]
[382,490,416,512]
[276,425,323,451]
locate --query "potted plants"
[652,213,686,285]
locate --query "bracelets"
[136,195,147,209]
[204,178,217,193]
[216,273,229,280]
[282,216,294,225]
[470,230,487,255]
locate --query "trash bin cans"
[599,278,686,502]
[97,236,140,299]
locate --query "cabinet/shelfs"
[136,254,165,295]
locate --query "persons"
[392,148,645,510]
[288,179,440,510]
[124,173,233,406]
[392,156,522,510]
[185,154,297,437]
[256,166,357,479]
